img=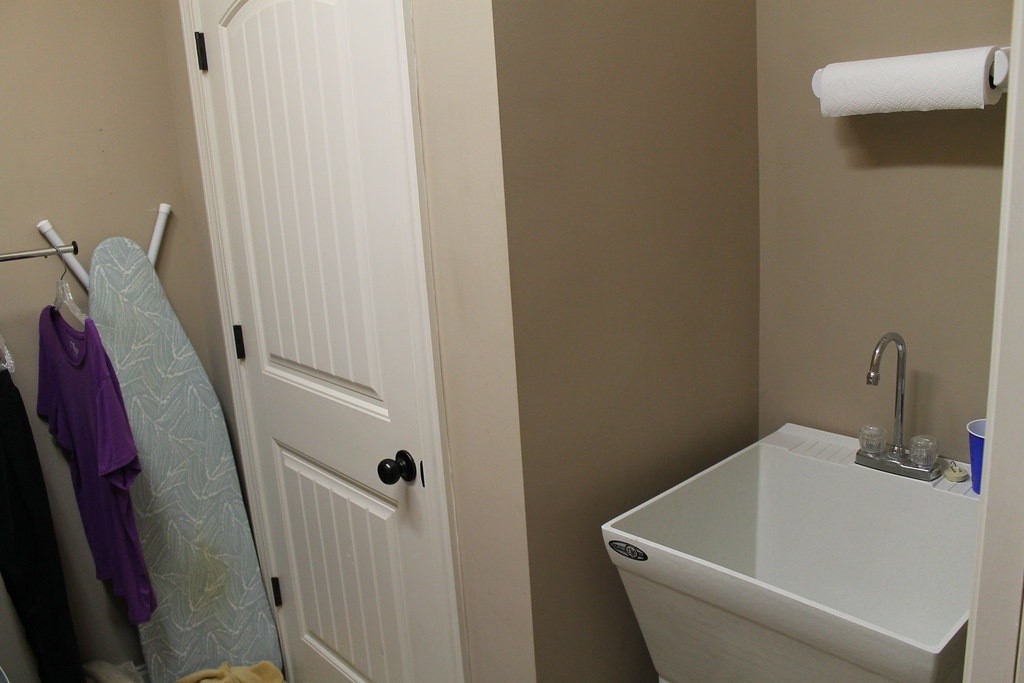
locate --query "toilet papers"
[819,42,1003,120]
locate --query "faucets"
[865,331,908,476]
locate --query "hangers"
[0,334,16,373]
[44,246,88,327]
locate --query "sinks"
[600,420,981,654]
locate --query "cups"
[966,418,987,495]
